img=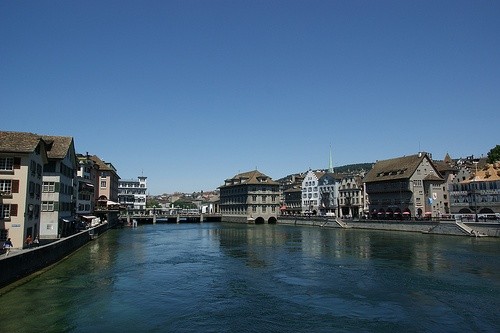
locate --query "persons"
[24,235,32,250]
[3,238,13,257]
[59,228,62,238]
[33,234,40,247]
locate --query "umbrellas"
[425,211,432,221]
